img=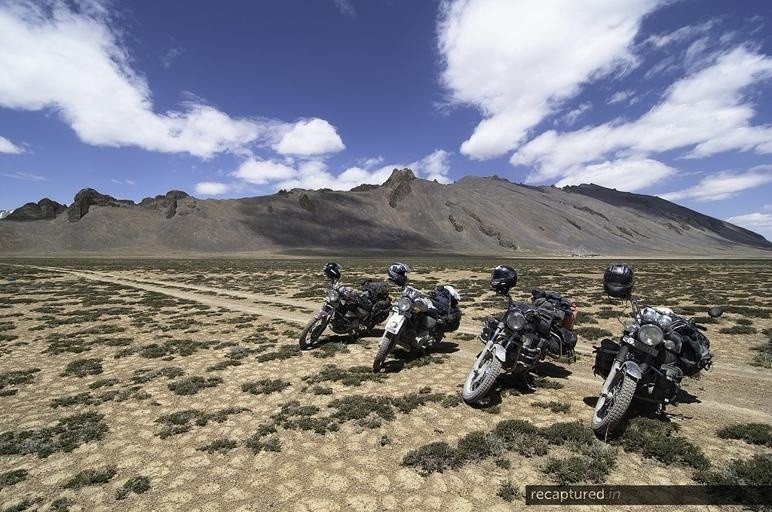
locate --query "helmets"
[388,261,411,285]
[603,265,634,297]
[491,265,518,289]
[323,261,342,279]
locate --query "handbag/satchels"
[683,344,711,377]
[371,297,392,324]
[551,327,577,355]
[440,310,461,331]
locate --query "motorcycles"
[373,260,467,378]
[298,258,390,352]
[462,265,578,407]
[585,262,728,435]
